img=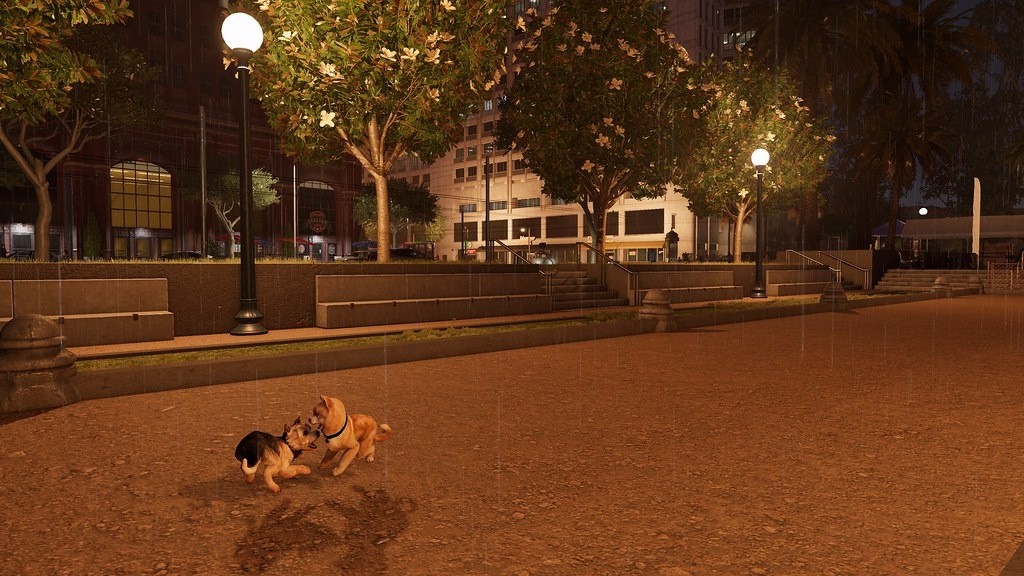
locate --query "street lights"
[752,149,770,297]
[222,10,266,336]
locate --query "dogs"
[308,394,392,477]
[233,416,320,494]
